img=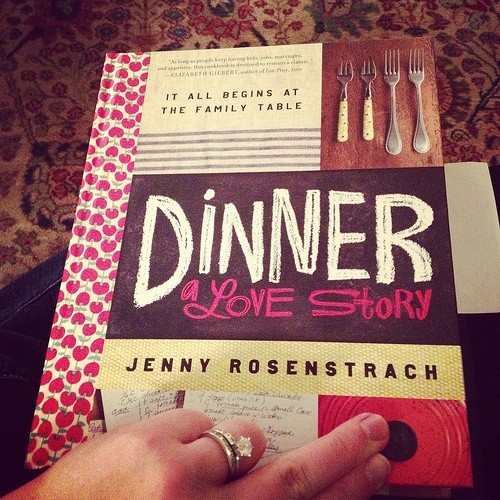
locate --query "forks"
[360,59,377,141]
[336,60,353,142]
[408,48,430,153]
[383,48,403,155]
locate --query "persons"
[2,407,395,500]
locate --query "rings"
[200,426,254,481]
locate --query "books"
[23,38,475,496]
